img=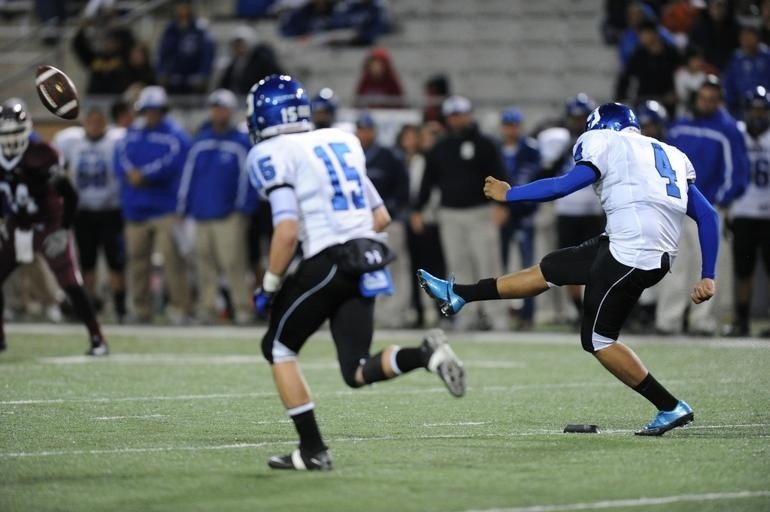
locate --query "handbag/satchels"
[334,239,391,271]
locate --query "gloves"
[43,228,68,259]
[253,285,274,313]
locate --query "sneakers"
[89,334,107,357]
[268,444,333,472]
[422,328,465,398]
[635,401,695,436]
[417,268,466,318]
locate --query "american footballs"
[35,66,79,119]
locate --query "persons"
[246,77,467,471]
[417,101,720,435]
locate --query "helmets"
[644,100,666,121]
[312,95,336,129]
[133,85,168,111]
[206,88,240,110]
[1,97,34,155]
[502,111,523,126]
[246,72,313,145]
[568,94,596,120]
[584,102,643,135]
[356,113,374,131]
[443,94,473,117]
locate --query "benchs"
[0,0,620,147]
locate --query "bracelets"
[261,269,285,294]
